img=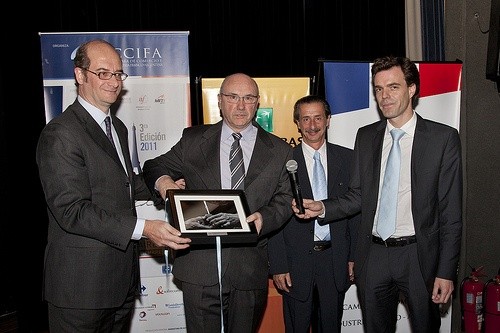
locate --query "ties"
[311,151,329,241]
[230,133,246,190]
[104,117,114,147]
[376,128,406,240]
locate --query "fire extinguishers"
[458,264,485,333]
[484,273,500,333]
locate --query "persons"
[265,96,354,333]
[142,74,296,332]
[290,60,461,333]
[36,39,191,333]
[182,211,241,230]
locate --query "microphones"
[285,160,305,215]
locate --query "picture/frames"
[166,189,259,245]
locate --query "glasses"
[86,69,128,80]
[222,94,259,103]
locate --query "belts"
[313,242,332,252]
[372,235,417,247]
